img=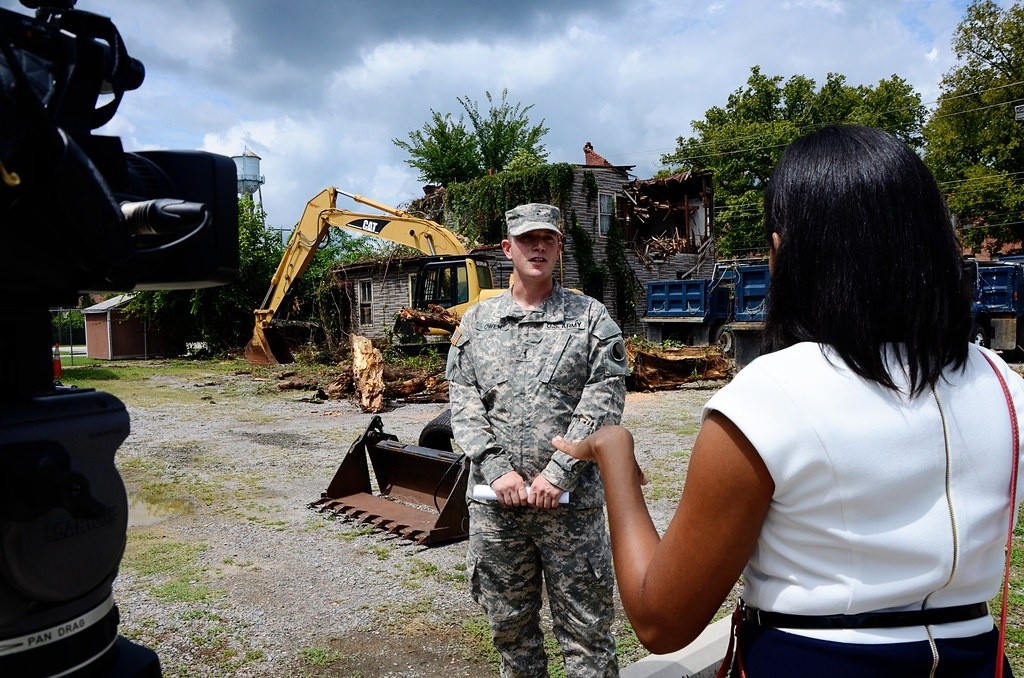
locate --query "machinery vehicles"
[242,185,512,368]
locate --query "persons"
[549,124,1023,677]
[443,200,630,678]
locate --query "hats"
[504,204,563,237]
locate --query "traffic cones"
[52,342,65,380]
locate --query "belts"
[736,596,989,629]
